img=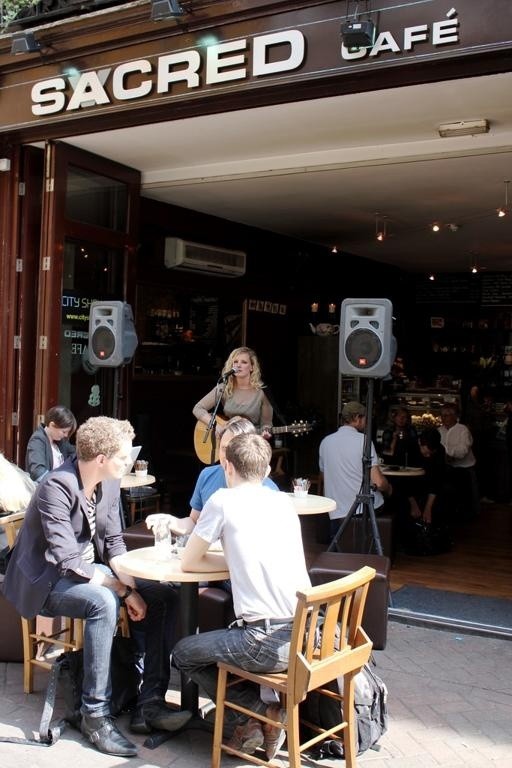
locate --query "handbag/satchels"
[55,636,143,732]
[400,516,448,557]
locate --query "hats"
[342,400,367,418]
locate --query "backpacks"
[314,644,393,762]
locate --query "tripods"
[324,377,393,608]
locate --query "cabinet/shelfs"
[387,389,463,426]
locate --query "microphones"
[223,368,238,378]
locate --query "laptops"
[123,446,142,476]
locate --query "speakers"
[89,301,138,368]
[338,298,396,378]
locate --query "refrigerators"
[338,367,359,426]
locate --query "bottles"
[154,516,171,561]
[274,435,283,448]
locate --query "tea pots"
[308,322,342,336]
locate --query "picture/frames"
[429,316,445,329]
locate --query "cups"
[134,469,148,477]
[294,490,308,498]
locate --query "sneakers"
[225,714,265,756]
[264,702,290,761]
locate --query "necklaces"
[235,384,251,390]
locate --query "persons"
[319,401,393,552]
[144,417,279,650]
[3,416,193,756]
[24,405,78,483]
[172,433,318,760]
[465,386,484,404]
[379,404,418,466]
[193,347,275,440]
[437,402,476,475]
[378,427,455,555]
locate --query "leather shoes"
[78,715,139,758]
[128,699,194,735]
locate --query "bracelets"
[260,422,273,427]
[119,585,132,601]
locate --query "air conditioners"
[164,237,248,282]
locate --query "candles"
[328,303,336,313]
[311,302,319,312]
